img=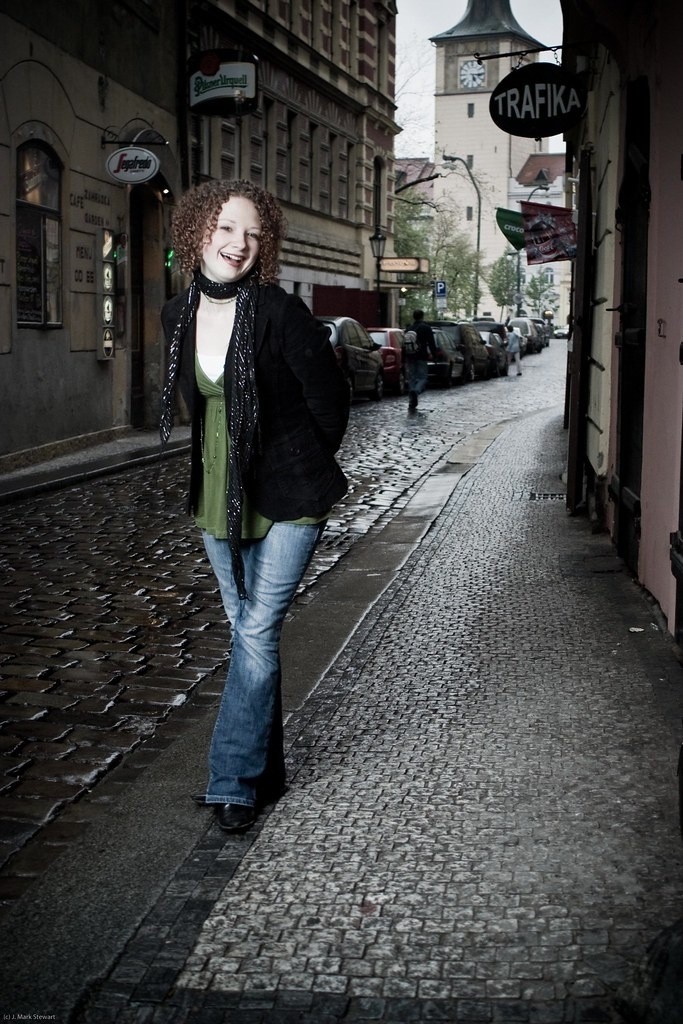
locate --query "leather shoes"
[216,803,255,832]
[192,793,215,806]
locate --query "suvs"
[313,314,385,404]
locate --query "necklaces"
[204,295,237,304]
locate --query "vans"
[417,320,494,379]
[467,314,549,376]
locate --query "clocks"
[460,60,486,89]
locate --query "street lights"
[442,154,482,322]
[369,222,388,292]
[514,185,549,317]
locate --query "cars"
[401,328,466,386]
[554,325,570,339]
[364,327,409,396]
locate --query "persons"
[400,310,438,409]
[505,325,522,376]
[159,179,351,829]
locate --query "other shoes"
[409,390,418,410]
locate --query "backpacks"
[403,323,425,354]
